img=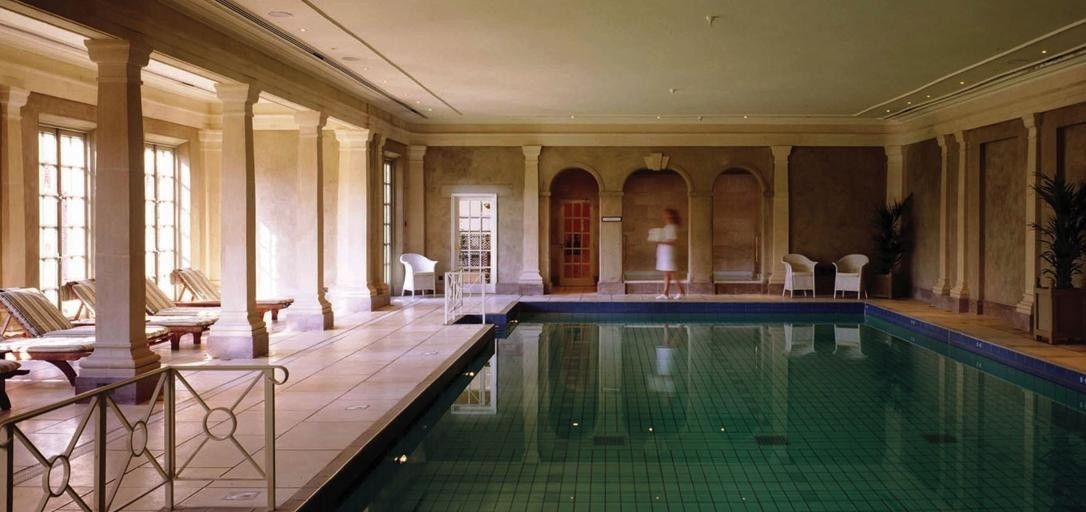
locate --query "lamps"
[484,203,490,209]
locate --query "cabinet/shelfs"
[487,252,490,273]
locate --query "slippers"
[657,293,684,299]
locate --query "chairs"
[0,359,30,410]
[781,323,817,359]
[170,269,294,321]
[831,254,870,300]
[780,254,819,299]
[832,323,868,364]
[0,335,95,386]
[400,253,440,299]
[61,279,220,350]
[0,287,174,346]
[145,277,221,316]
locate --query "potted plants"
[870,192,913,299]
[1025,171,1086,344]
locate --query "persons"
[645,207,687,301]
[652,322,686,407]
